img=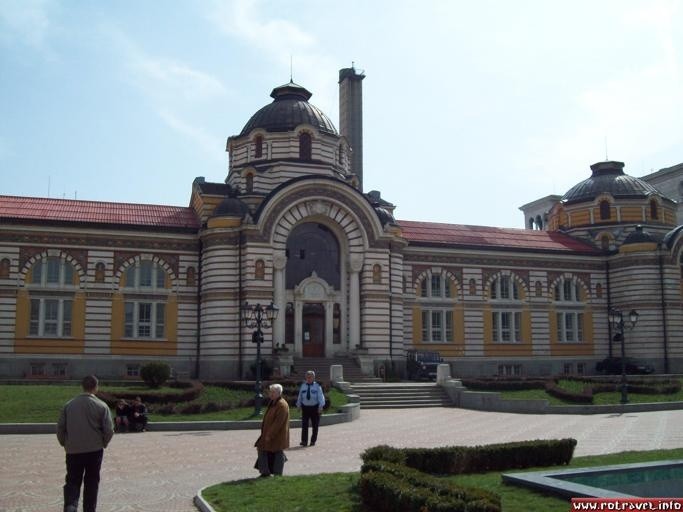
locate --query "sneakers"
[300,442,315,446]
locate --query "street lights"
[240,301,279,417]
[608,307,639,404]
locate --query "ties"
[307,384,310,400]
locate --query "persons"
[56,373,113,512]
[113,396,131,432]
[128,396,147,433]
[252,383,289,476]
[295,369,325,446]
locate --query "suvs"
[403,348,448,381]
